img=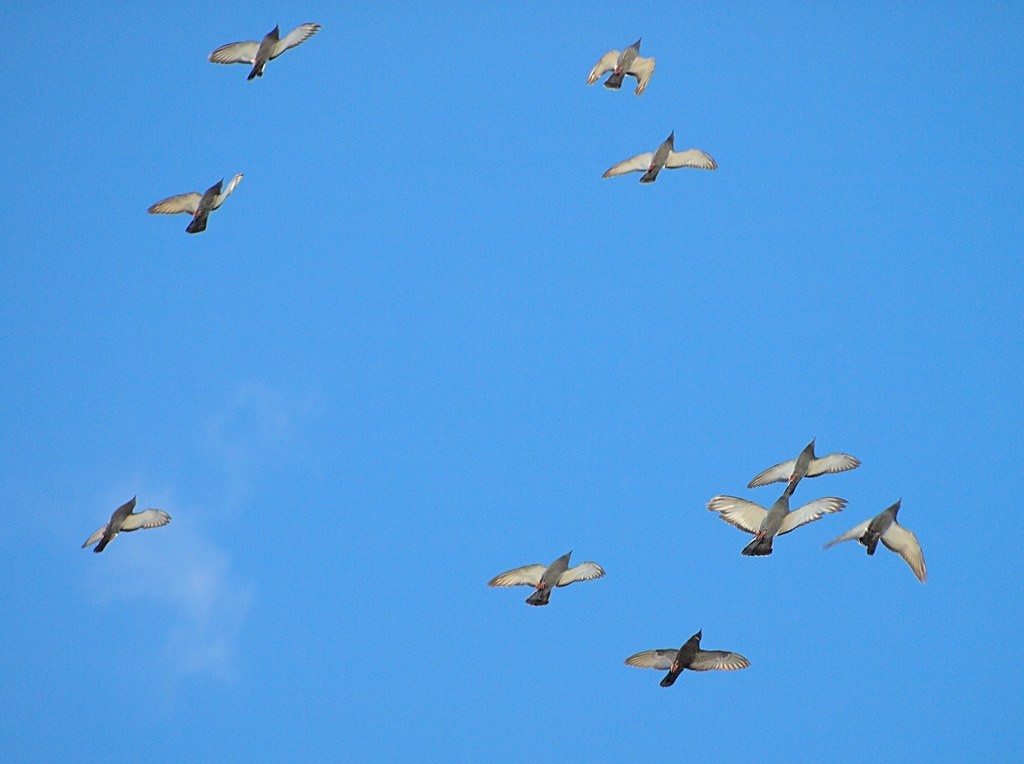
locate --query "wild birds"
[487,436,931,687]
[80,495,173,553]
[145,173,244,234]
[209,22,321,81]
[586,37,656,97]
[602,129,718,184]
